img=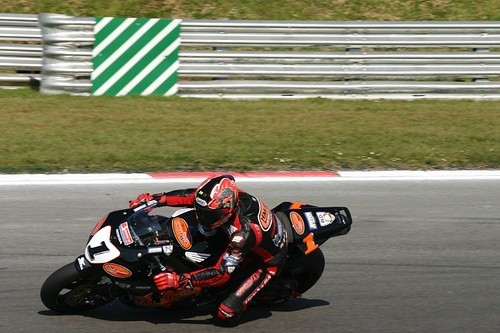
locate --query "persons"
[127,174,303,323]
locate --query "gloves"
[129,193,153,214]
[154,273,177,290]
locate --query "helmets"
[195,175,239,230]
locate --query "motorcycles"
[39,198,352,317]
